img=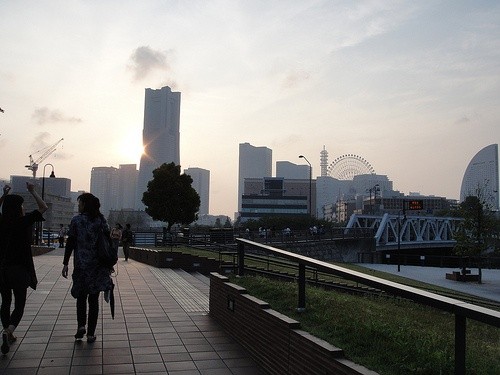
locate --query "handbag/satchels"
[96,231,119,267]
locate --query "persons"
[58,223,66,248]
[245,224,325,241]
[0,181,48,353]
[110,223,132,261]
[61,193,110,343]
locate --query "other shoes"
[86,335,97,342]
[75,327,86,338]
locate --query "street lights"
[39,164,56,245]
[298,155,312,216]
[369,184,380,228]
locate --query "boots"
[1,324,15,355]
[8,330,17,346]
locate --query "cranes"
[24,137,65,176]
